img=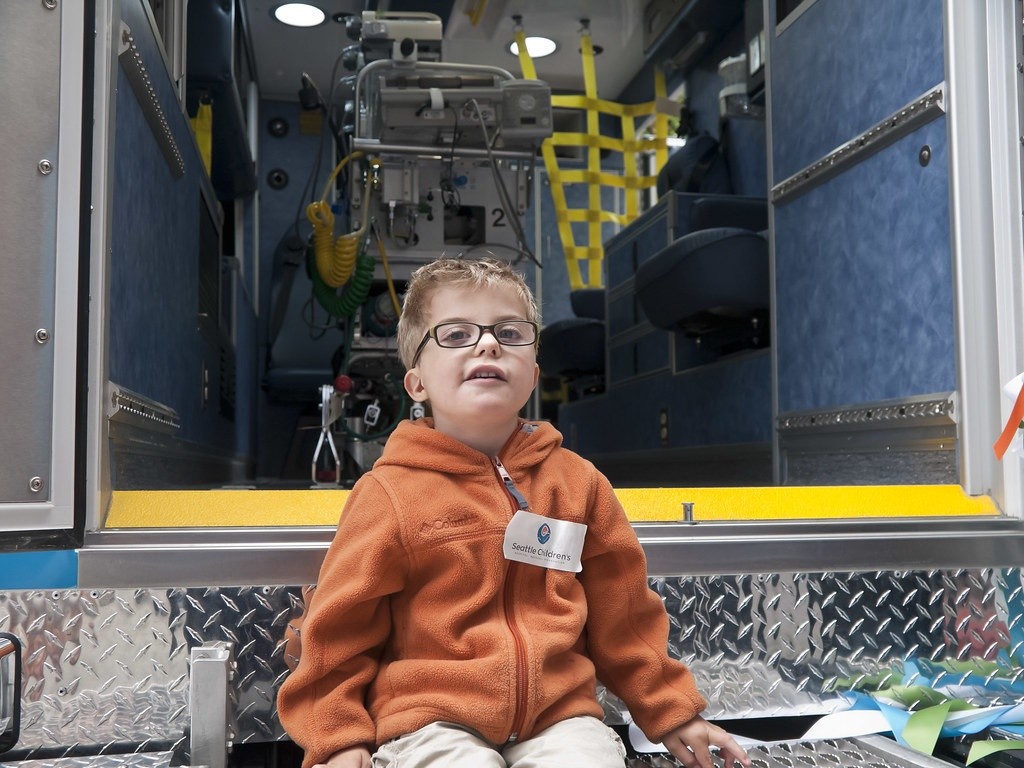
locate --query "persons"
[277,251,753,768]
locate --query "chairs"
[634,194,768,354]
[536,290,606,392]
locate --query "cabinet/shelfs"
[601,192,766,394]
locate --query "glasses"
[412,320,538,368]
